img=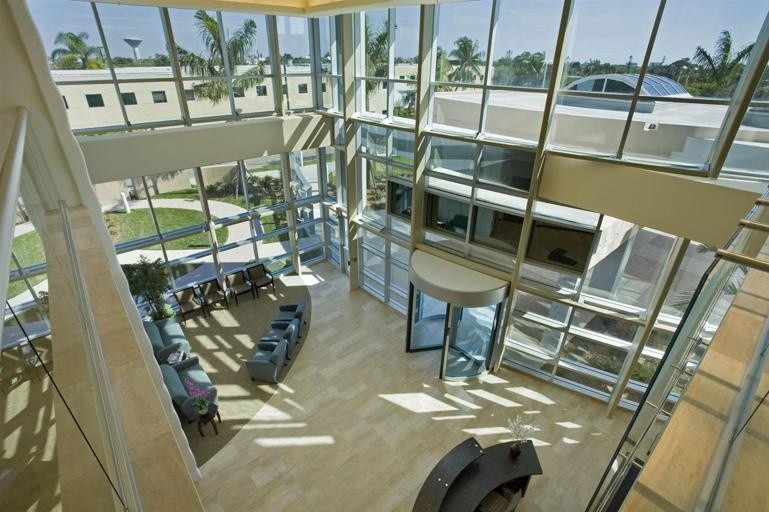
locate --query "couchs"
[143,317,217,423]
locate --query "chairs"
[245,300,311,384]
[173,264,276,324]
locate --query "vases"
[511,441,523,460]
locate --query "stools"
[193,403,223,437]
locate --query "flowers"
[507,414,542,446]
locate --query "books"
[167,350,184,364]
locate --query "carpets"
[177,272,312,469]
